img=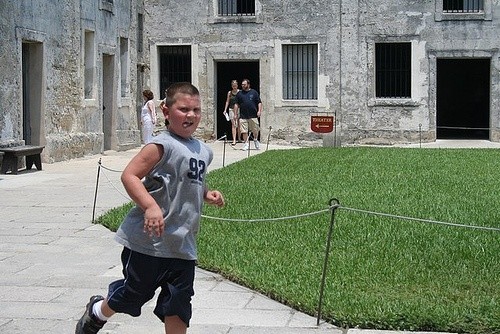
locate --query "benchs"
[0,145,46,175]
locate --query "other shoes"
[255,140,260,148]
[231,142,236,146]
[243,143,249,150]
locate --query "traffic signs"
[310,115,333,134]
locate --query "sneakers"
[75,295,107,334]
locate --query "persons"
[234,79,262,150]
[75,82,224,334]
[223,80,245,146]
